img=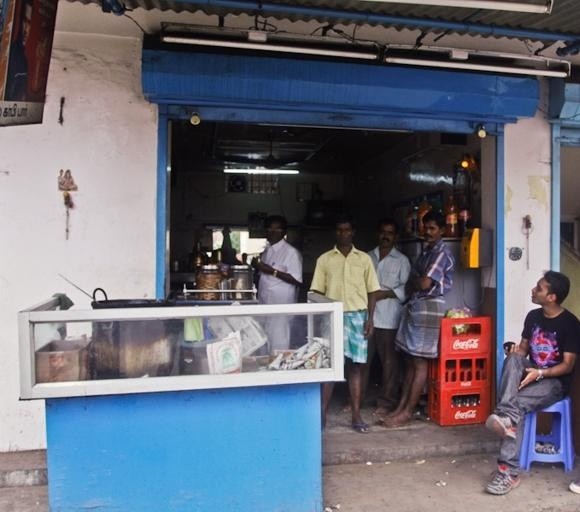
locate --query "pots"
[88,288,183,341]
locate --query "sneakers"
[486,414,517,440]
[485,466,520,494]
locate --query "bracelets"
[535,370,543,382]
[273,270,277,277]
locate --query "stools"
[520,397,576,476]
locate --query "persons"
[289,240,315,295]
[241,252,250,265]
[479,267,580,496]
[231,248,241,265]
[309,219,379,435]
[4,0,34,102]
[339,221,411,419]
[58,168,77,191]
[251,215,303,353]
[376,210,456,429]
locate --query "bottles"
[409,193,472,238]
[451,397,480,409]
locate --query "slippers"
[352,418,369,432]
